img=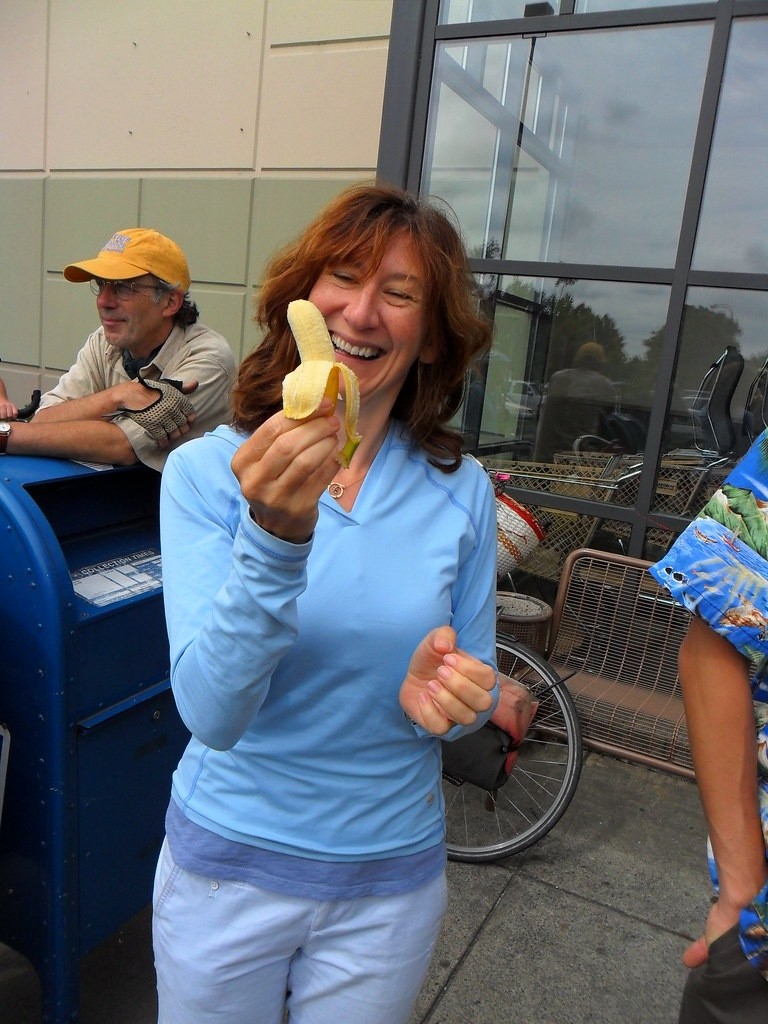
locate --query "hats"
[575,343,606,364]
[64,228,191,297]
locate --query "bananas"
[280,299,364,468]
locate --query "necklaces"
[325,473,363,497]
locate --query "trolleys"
[549,447,734,550]
[478,450,644,594]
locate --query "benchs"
[509,547,760,782]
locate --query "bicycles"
[443,453,584,865]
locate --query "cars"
[669,396,709,449]
[502,394,534,420]
[506,380,546,413]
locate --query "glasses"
[90,277,164,298]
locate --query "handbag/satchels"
[442,672,537,790]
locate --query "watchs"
[0,419,11,455]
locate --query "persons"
[157,182,503,1024]
[0,227,238,466]
[0,377,18,421]
[645,424,768,1024]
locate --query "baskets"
[497,484,545,583]
[493,590,553,679]
